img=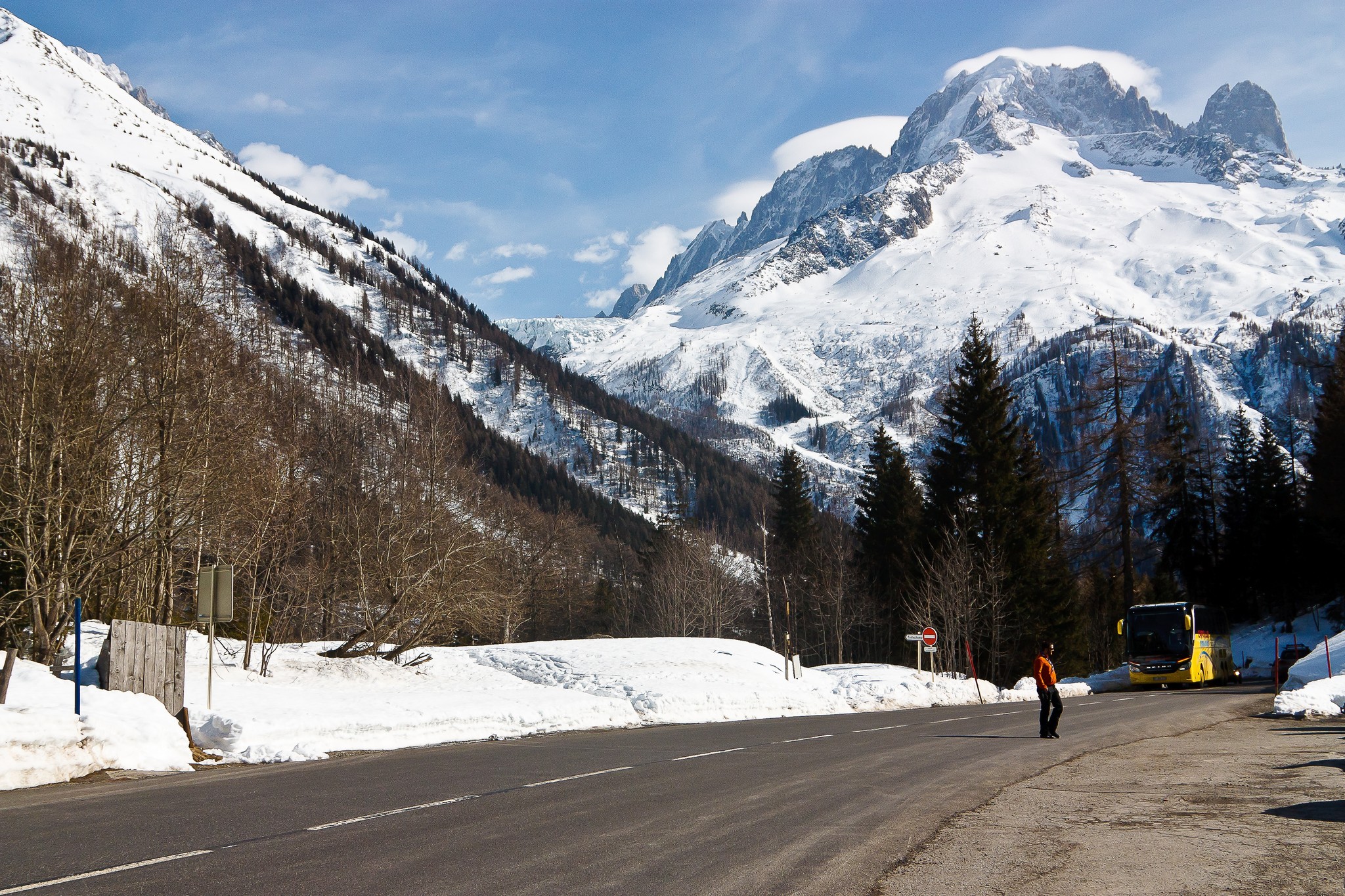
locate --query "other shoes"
[1040,734,1054,739]
[1049,731,1060,738]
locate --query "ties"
[1042,654,1054,673]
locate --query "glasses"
[1046,648,1054,650]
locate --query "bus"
[1117,602,1235,692]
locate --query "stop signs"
[922,627,938,646]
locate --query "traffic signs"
[905,634,923,641]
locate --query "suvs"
[1268,643,1312,683]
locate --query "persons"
[1033,641,1063,739]
[1146,635,1153,644]
[1175,633,1185,645]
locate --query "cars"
[1233,662,1242,685]
